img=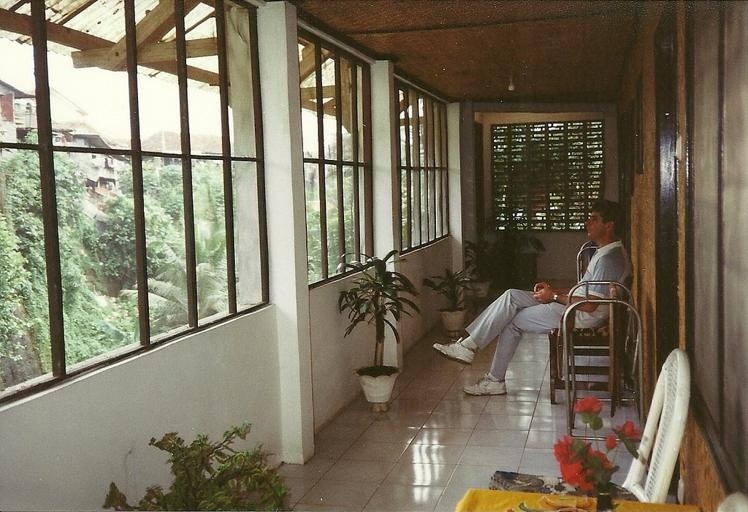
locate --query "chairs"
[549,274,633,404]
[489,349,691,504]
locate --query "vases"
[596,493,614,512]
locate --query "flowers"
[553,396,643,492]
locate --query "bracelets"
[552,294,558,302]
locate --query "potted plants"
[464,239,492,297]
[423,267,474,336]
[335,249,422,403]
[491,206,546,290]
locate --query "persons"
[433,200,632,396]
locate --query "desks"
[455,488,701,512]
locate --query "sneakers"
[463,373,507,397]
[432,341,476,366]
